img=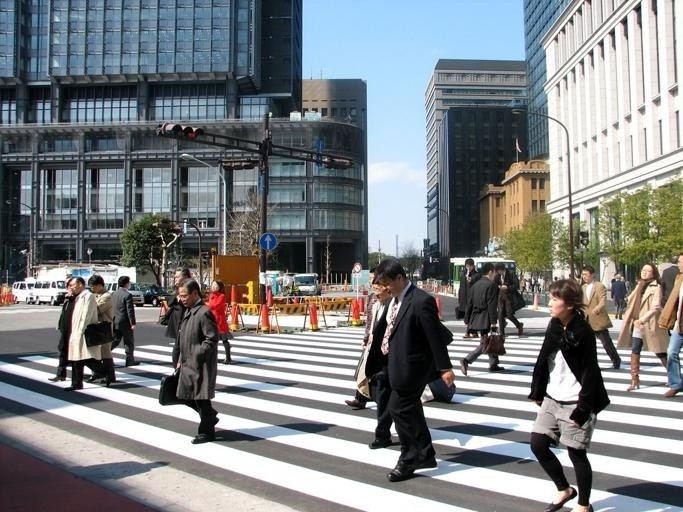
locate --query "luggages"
[429,378,456,403]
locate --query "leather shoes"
[191,432,216,444]
[613,358,620,370]
[126,361,140,366]
[370,437,392,449]
[48,373,115,391]
[346,400,367,408]
[388,456,437,482]
[663,389,678,397]
[545,487,577,511]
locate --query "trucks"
[292,272,322,296]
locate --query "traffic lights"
[161,122,205,140]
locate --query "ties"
[380,303,399,355]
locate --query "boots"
[627,353,640,391]
[223,342,231,363]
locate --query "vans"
[106,282,171,307]
[12,280,68,306]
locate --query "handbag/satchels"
[160,307,173,325]
[455,307,464,320]
[85,321,114,347]
[159,368,186,405]
[482,329,505,354]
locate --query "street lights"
[510,107,574,282]
[161,219,203,292]
[6,199,35,277]
[182,153,227,256]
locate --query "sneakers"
[460,358,469,375]
[518,323,523,335]
[464,331,479,338]
[488,367,505,372]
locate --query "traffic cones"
[532,293,540,310]
[230,284,365,334]
[435,296,445,321]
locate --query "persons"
[351,258,456,482]
[579,254,683,397]
[457,259,524,374]
[520,276,537,291]
[48,268,234,445]
[526,279,610,512]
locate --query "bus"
[448,257,516,296]
